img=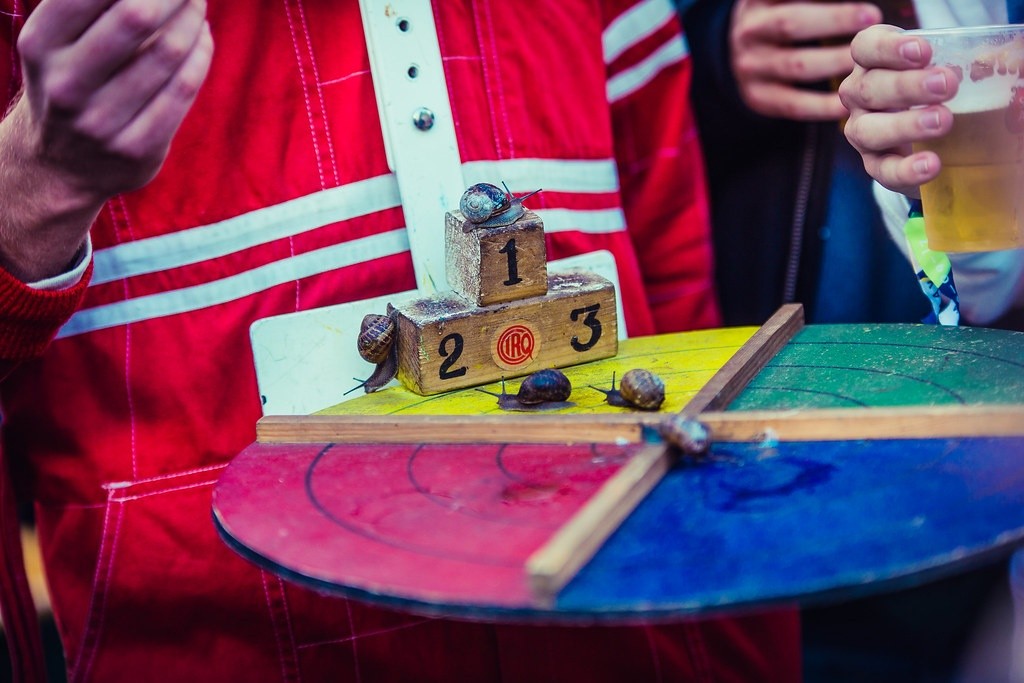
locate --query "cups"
[896,23,1024,253]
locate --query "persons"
[0,0,800,683]
[668,0,1024,683]
